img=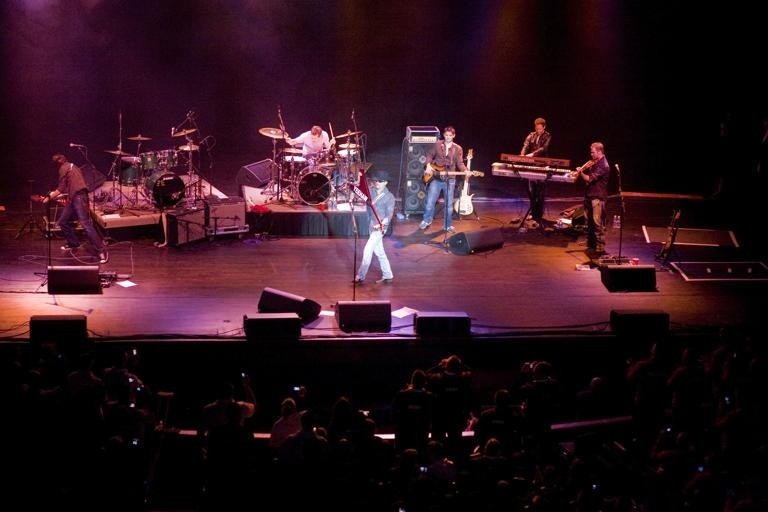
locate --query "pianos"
[491,152,575,186]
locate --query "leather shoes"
[351,277,362,283]
[376,279,393,283]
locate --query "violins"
[567,158,597,179]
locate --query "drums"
[299,171,334,204]
[335,150,360,168]
[283,148,303,155]
[156,148,180,173]
[120,165,141,184]
[142,152,161,173]
[284,158,306,186]
[338,143,359,160]
[147,171,184,207]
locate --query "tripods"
[101,145,205,217]
[33,205,50,286]
[261,139,361,210]
[14,180,43,240]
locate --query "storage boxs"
[207,197,246,237]
[156,208,209,246]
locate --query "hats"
[369,170,393,181]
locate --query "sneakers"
[60,244,73,250]
[441,225,455,233]
[419,220,431,229]
[99,251,110,264]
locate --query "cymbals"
[105,149,130,156]
[336,131,361,140]
[122,158,143,164]
[179,146,201,151]
[129,136,151,141]
[259,127,289,140]
[173,129,197,138]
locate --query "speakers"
[203,195,250,241]
[243,312,302,340]
[258,287,321,324]
[236,158,278,187]
[165,206,208,247]
[414,312,470,337]
[610,309,669,335]
[401,140,435,215]
[449,227,504,256]
[47,266,103,294]
[335,301,392,333]
[30,315,87,340]
[600,264,656,293]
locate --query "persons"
[350,170,395,285]
[39,153,109,265]
[285,125,334,159]
[519,117,552,220]
[0,346,767,511]
[418,127,468,234]
[571,141,612,260]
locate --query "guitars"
[454,149,474,217]
[423,163,484,182]
[347,169,383,232]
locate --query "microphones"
[614,164,621,174]
[440,143,445,155]
[70,143,84,147]
